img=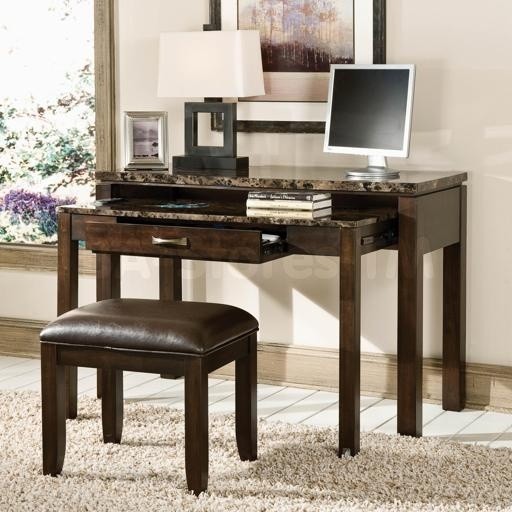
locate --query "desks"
[52,165,472,458]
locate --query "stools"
[38,294,260,496]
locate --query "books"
[245,190,332,220]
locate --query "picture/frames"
[207,0,385,137]
[120,110,168,170]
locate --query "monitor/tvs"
[323,63,416,177]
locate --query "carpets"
[2,383,511,509]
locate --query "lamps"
[153,28,266,178]
